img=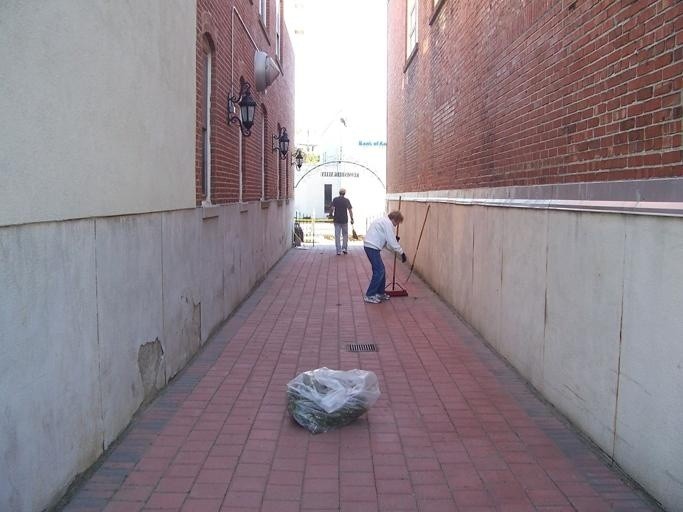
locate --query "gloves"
[402,253,406,263]
[396,236,400,241]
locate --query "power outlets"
[291,159,303,169]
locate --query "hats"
[339,189,345,193]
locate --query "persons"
[363,211,406,304]
[330,188,354,255]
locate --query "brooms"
[385,196,409,297]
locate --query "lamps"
[228,76,256,137]
[291,147,304,162]
[271,127,289,160]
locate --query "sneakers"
[364,295,381,304]
[376,293,390,300]
[342,248,347,254]
[336,252,341,255]
